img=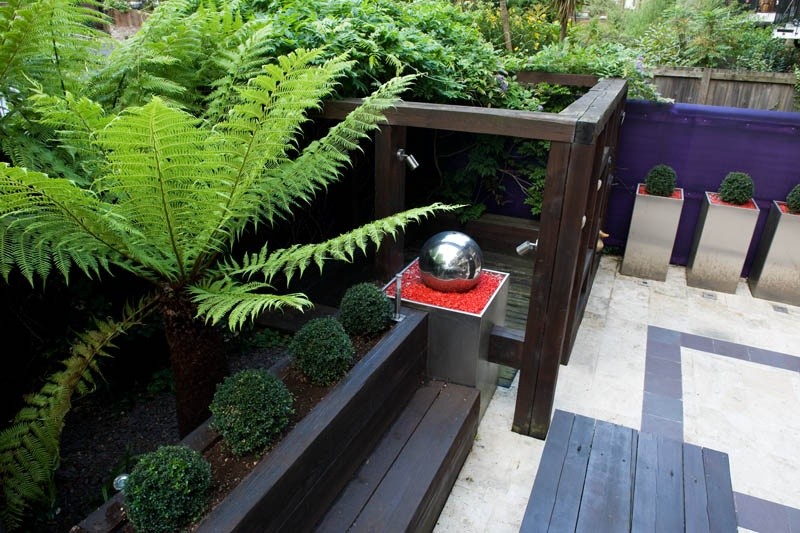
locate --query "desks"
[516,410,739,532]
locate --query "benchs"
[194,309,485,533]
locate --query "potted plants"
[686,170,761,295]
[620,164,685,282]
[746,183,800,307]
